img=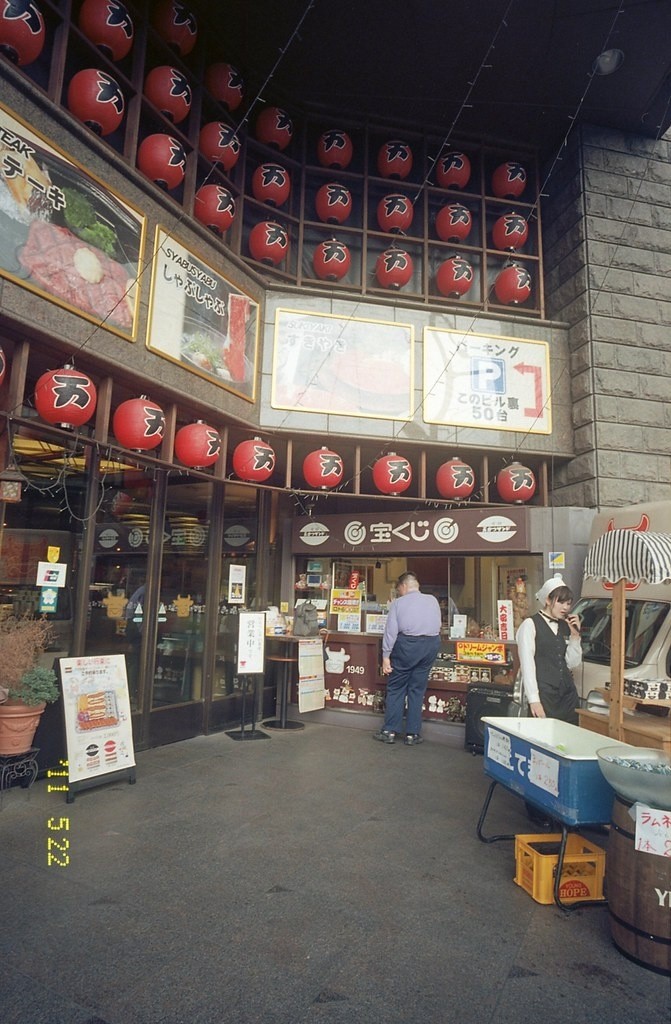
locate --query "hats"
[535,573,567,608]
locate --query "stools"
[1,747,41,812]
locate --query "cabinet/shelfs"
[375,640,520,692]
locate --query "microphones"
[566,616,580,634]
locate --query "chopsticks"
[229,294,260,306]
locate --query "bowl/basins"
[181,316,252,390]
[596,745,671,809]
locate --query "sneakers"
[374,730,396,743]
[405,733,423,745]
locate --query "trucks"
[507,499,671,718]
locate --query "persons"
[515,578,585,727]
[373,570,442,744]
[125,585,146,703]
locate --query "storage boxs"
[514,833,607,904]
[481,717,637,827]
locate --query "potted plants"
[0,607,60,754]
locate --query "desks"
[262,634,321,731]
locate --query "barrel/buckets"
[605,790,671,978]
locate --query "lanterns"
[232,436,276,483]
[175,419,222,471]
[303,447,344,491]
[496,460,535,504]
[112,395,166,453]
[111,493,133,517]
[35,365,97,430]
[372,452,412,496]
[436,457,475,501]
[1,0,533,307]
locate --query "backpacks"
[293,599,319,636]
